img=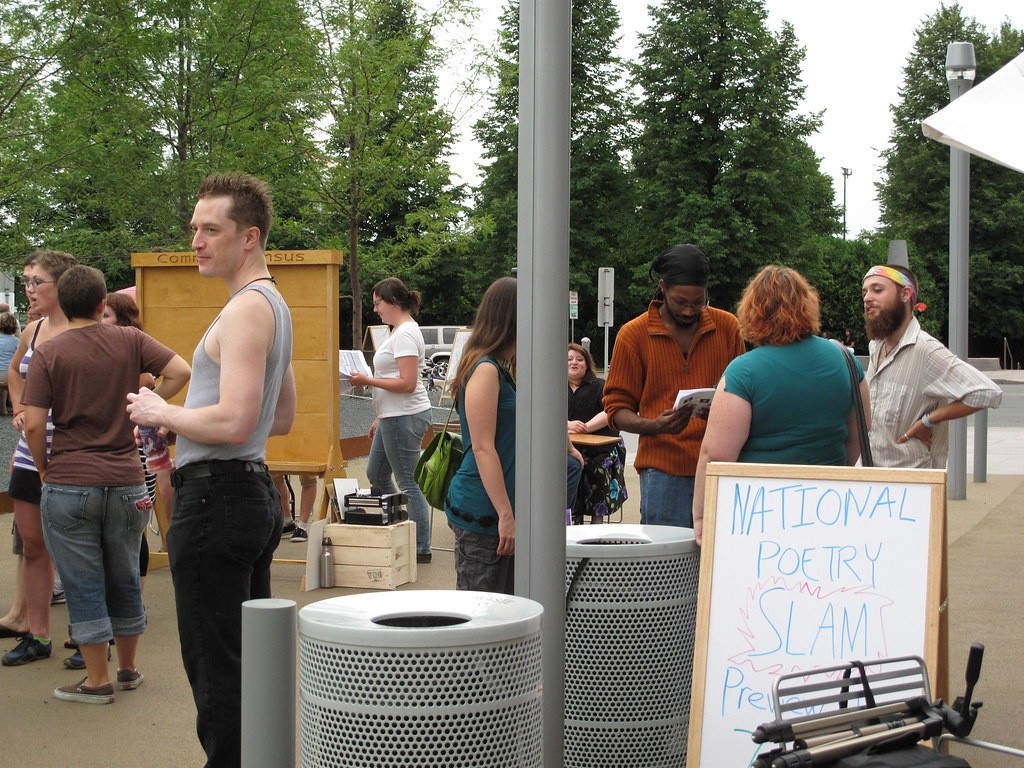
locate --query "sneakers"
[117,667,144,689]
[54,676,115,704]
[2,633,52,666]
[63,641,111,668]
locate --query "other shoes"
[415,553,432,564]
[63,638,115,649]
[281,521,296,539]
[290,527,309,542]
[50,590,66,605]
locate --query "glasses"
[22,278,57,288]
[372,298,384,308]
[27,312,42,318]
[665,288,710,311]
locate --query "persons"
[349,277,432,564]
[692,264,872,547]
[445,276,584,595]
[841,328,854,348]
[821,331,827,339]
[855,264,1003,468]
[19,264,193,703]
[127,171,296,768]
[0,246,317,668]
[567,343,629,526]
[602,243,746,529]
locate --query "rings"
[904,433,909,440]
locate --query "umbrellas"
[921,51,1024,173]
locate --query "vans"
[419,325,473,380]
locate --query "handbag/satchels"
[414,431,465,511]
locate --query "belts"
[169,460,270,489]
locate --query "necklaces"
[223,277,277,308]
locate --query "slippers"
[0,623,31,638]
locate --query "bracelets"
[11,409,24,417]
[922,413,936,427]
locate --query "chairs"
[428,423,462,552]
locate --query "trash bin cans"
[299,589,543,768]
[560,524,701,768]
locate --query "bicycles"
[420,357,445,391]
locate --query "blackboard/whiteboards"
[686,461,949,768]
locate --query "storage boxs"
[325,520,418,589]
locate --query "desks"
[568,434,623,524]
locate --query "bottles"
[320,537,335,588]
[137,420,173,472]
